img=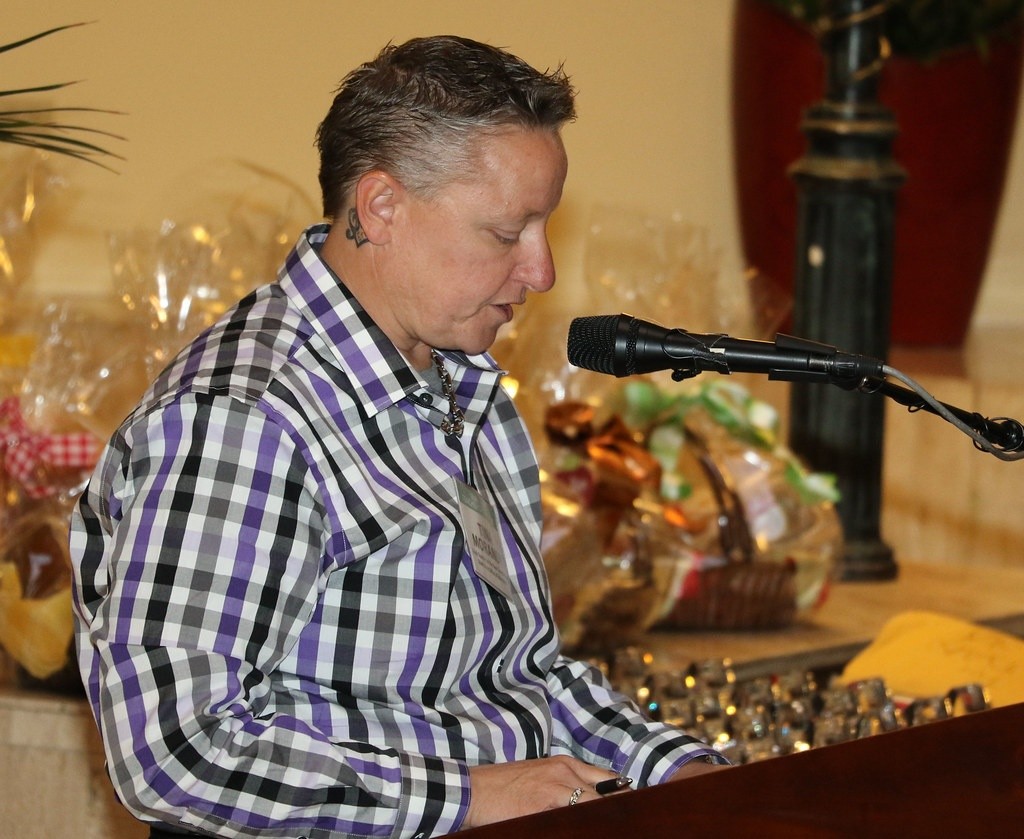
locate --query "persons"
[71,37,732,839]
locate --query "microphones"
[567,312,886,382]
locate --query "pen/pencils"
[588,777,633,795]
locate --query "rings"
[568,787,585,806]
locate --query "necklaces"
[409,347,465,437]
